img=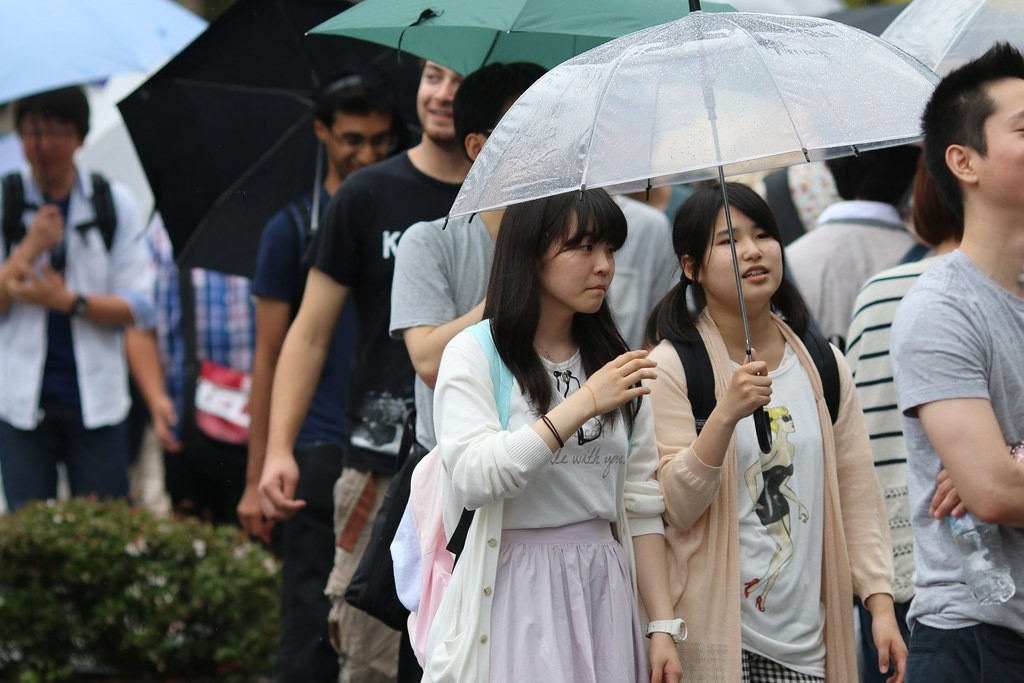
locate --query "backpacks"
[389,316,633,669]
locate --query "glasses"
[326,128,400,154]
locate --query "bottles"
[947,486,1015,607]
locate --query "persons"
[0,0,1024,683]
